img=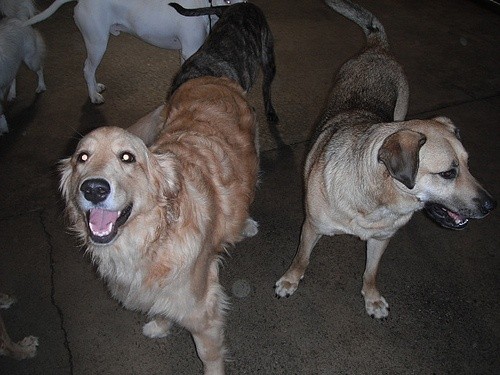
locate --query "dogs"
[20,0,249,104]
[168,1,279,125]
[0,0,47,136]
[48,76,267,375]
[272,0,497,321]
